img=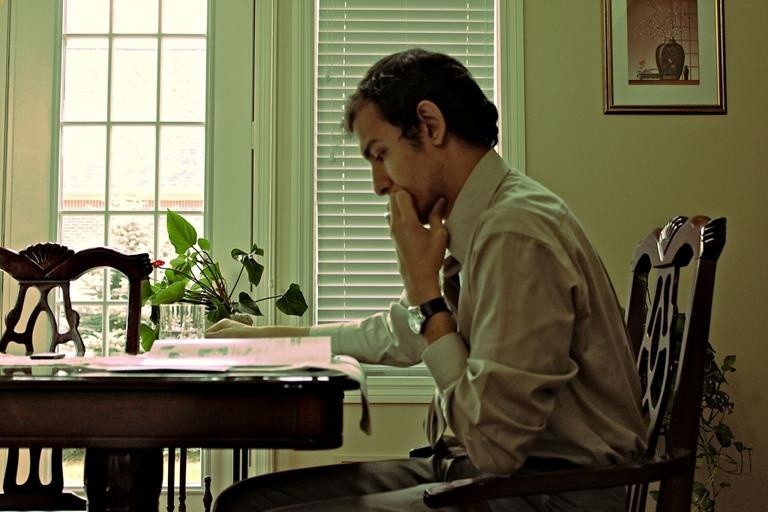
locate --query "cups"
[159,304,206,340]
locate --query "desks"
[1,365,363,511]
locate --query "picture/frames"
[601,0,727,115]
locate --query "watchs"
[408,296,449,335]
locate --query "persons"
[202,48,650,511]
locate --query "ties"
[422,248,467,458]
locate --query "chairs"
[0,242,159,511]
[408,212,729,511]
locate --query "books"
[147,336,333,368]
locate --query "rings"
[382,211,392,220]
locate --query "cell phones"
[31,353,66,359]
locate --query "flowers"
[138,207,310,352]
